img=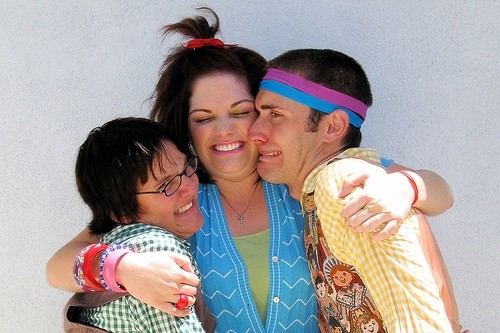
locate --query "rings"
[176,295,189,309]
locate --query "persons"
[44,8,453,333]
[249,48,463,333]
[63,117,216,333]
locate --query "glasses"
[137,155,197,197]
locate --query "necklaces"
[219,181,259,223]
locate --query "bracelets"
[396,170,418,205]
[72,244,133,292]
